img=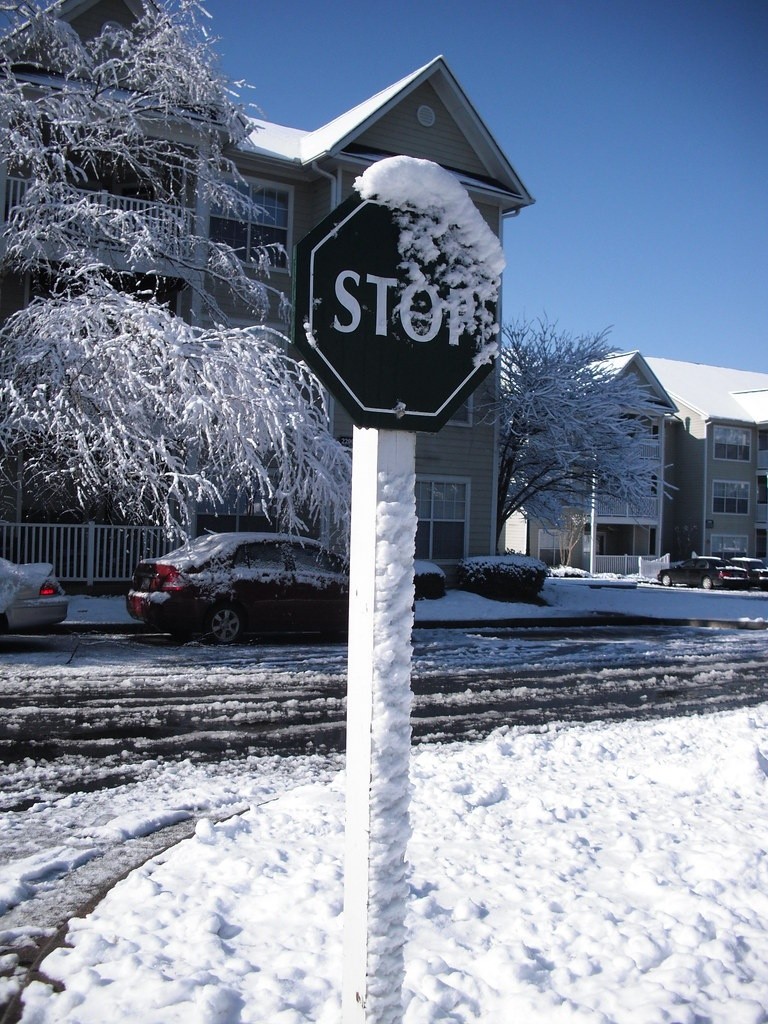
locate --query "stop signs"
[287,159,504,437]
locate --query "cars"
[0,560,70,630]
[728,557,767,587]
[658,555,748,590]
[124,532,415,646]
